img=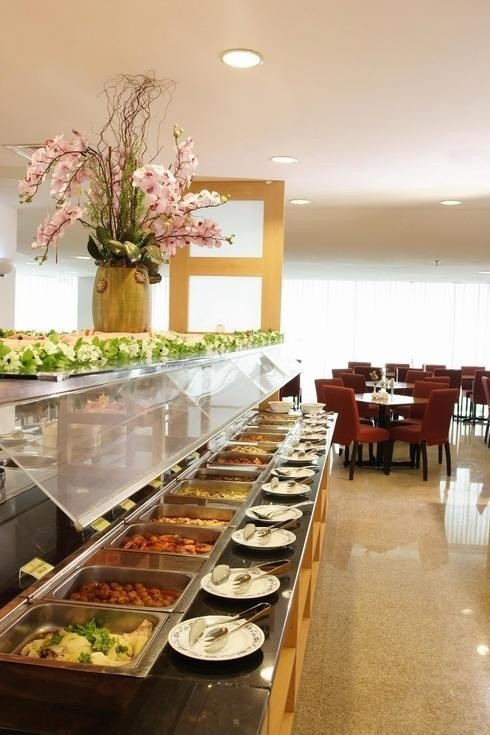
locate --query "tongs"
[211,559,291,590]
[189,603,272,654]
[256,518,297,544]
[260,410,329,495]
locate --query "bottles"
[372,373,395,400]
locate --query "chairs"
[313,356,490,482]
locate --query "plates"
[265,409,293,414]
[201,568,281,600]
[247,505,303,523]
[168,615,266,661]
[232,527,296,551]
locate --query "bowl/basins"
[268,401,294,412]
[300,403,326,412]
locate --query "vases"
[91,266,153,335]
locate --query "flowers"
[19,62,234,264]
